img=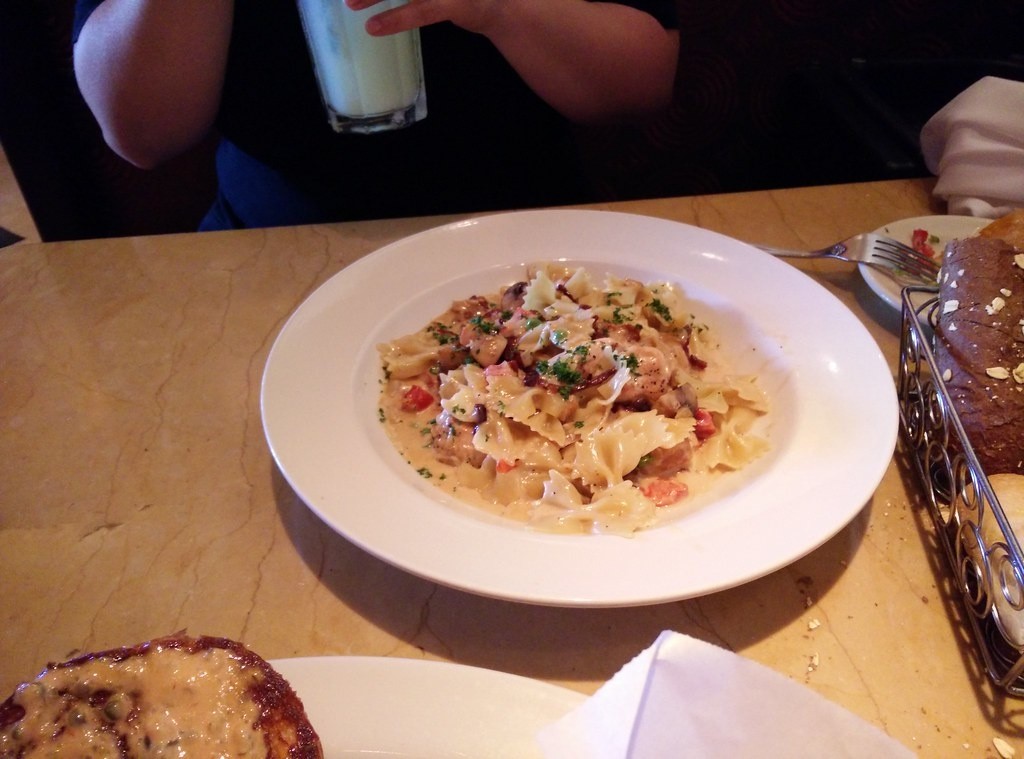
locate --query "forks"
[745,232,941,283]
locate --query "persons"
[73,0,682,232]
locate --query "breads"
[0,627,324,759]
[956,472,1024,649]
[930,206,1024,485]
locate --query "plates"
[260,209,904,609]
[857,215,999,325]
[260,656,592,759]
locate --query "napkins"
[545,626,916,759]
[920,76,1024,218]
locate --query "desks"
[0,177,1024,759]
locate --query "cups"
[296,0,427,134]
